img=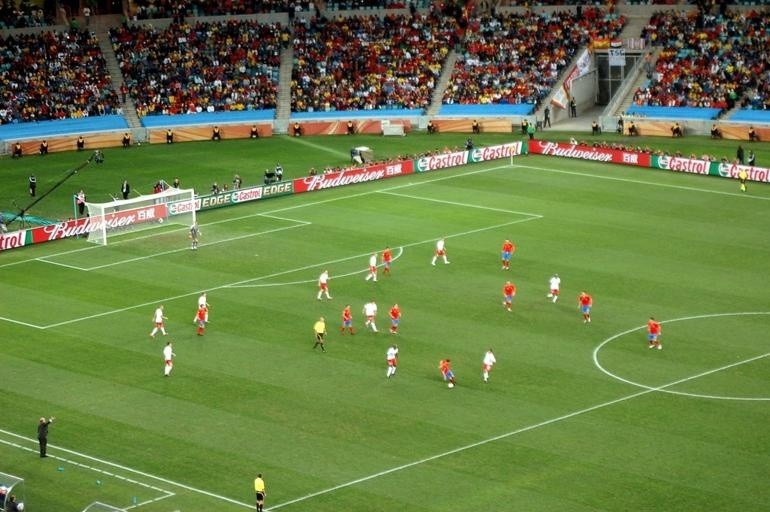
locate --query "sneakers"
[649,345,655,348]
[162,333,168,336]
[454,383,456,386]
[431,262,436,265]
[317,298,323,301]
[373,279,379,282]
[508,308,512,311]
[552,299,556,302]
[322,350,327,353]
[351,332,354,335]
[200,334,203,336]
[505,267,510,270]
[391,374,395,376]
[192,320,195,325]
[584,320,587,323]
[192,246,194,249]
[194,246,198,249]
[658,344,662,350]
[257,510,260,512]
[150,334,155,338]
[327,296,333,300]
[389,328,393,333]
[588,318,590,322]
[546,294,549,298]
[386,376,390,379]
[205,321,210,324]
[166,374,169,377]
[445,261,450,264]
[484,379,487,383]
[393,331,397,334]
[365,322,369,328]
[502,265,505,269]
[40,455,46,458]
[375,331,380,333]
[197,333,200,335]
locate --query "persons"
[737,146,745,165]
[78,189,86,215]
[37,415,56,457]
[347,121,354,136]
[381,247,393,276]
[167,129,173,143]
[275,163,283,181]
[618,117,623,133]
[632,1,770,111]
[192,292,211,324]
[121,180,129,199]
[342,304,355,335]
[121,1,290,18]
[317,270,333,300]
[365,252,379,282]
[571,97,576,118]
[361,299,379,333]
[290,0,402,14]
[739,169,748,192]
[711,124,720,136]
[430,235,451,265]
[123,133,130,146]
[150,305,169,338]
[12,143,23,158]
[0,28,107,120]
[427,121,433,135]
[255,473,267,512]
[472,120,478,133]
[439,359,456,386]
[213,181,218,194]
[576,0,628,47]
[443,67,566,104]
[107,18,291,118]
[577,290,592,323]
[519,0,576,65]
[198,304,208,336]
[629,121,635,136]
[77,136,84,150]
[671,123,682,136]
[547,273,561,304]
[522,119,528,134]
[50,0,106,67]
[41,140,48,155]
[250,125,259,138]
[154,178,165,192]
[212,126,220,140]
[290,13,457,112]
[592,121,598,130]
[565,138,731,165]
[163,341,176,376]
[313,317,328,353]
[293,122,301,136]
[482,349,496,382]
[748,126,754,141]
[324,143,459,174]
[0,484,20,512]
[454,0,519,65]
[309,168,317,175]
[748,150,755,166]
[174,178,179,189]
[385,344,399,379]
[648,317,663,350]
[527,123,536,139]
[232,175,241,189]
[544,106,551,128]
[0,0,48,28]
[501,239,515,269]
[29,173,36,196]
[189,220,202,250]
[388,304,402,334]
[465,136,472,149]
[502,280,516,312]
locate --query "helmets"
[17,503,24,511]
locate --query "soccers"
[448,383,454,388]
